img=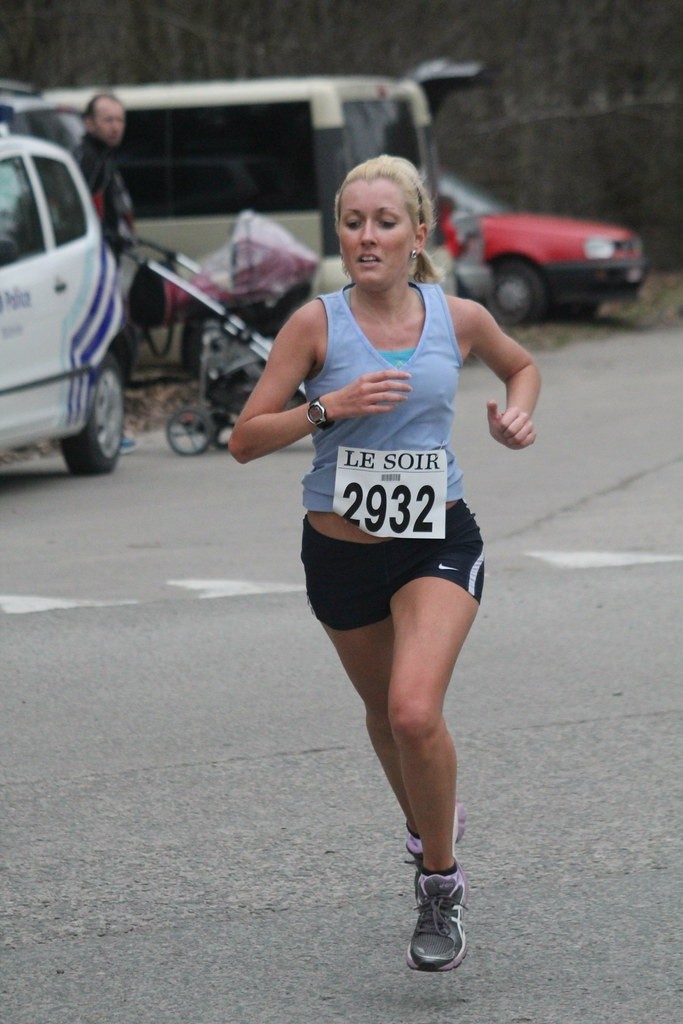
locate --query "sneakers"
[404,803,465,912]
[407,856,470,971]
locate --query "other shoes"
[120,439,137,454]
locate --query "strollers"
[115,206,323,459]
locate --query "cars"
[0,124,131,479]
[432,169,647,333]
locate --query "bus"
[0,70,442,402]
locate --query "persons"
[73,93,135,454]
[228,156,542,973]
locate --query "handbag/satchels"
[128,258,187,357]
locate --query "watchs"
[306,397,335,431]
